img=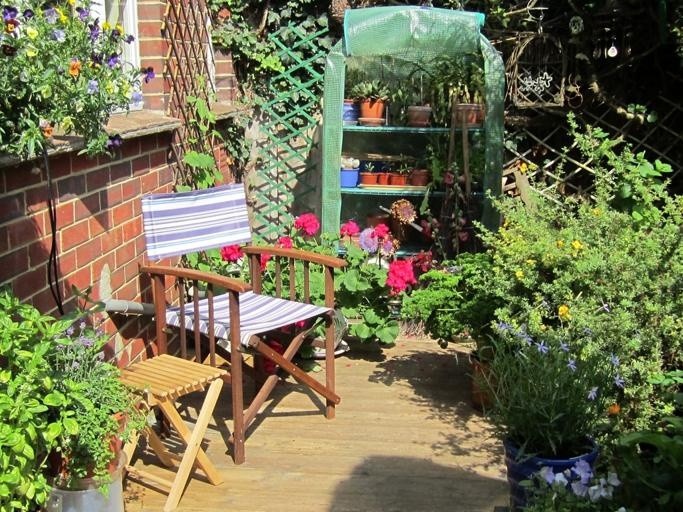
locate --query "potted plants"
[340,56,487,193]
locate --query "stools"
[109,354,232,508]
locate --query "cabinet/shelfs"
[322,5,508,307]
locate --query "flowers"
[218,199,435,335]
[0,0,157,165]
[471,300,645,510]
[38,283,151,497]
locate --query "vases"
[49,410,128,489]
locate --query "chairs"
[137,180,350,465]
